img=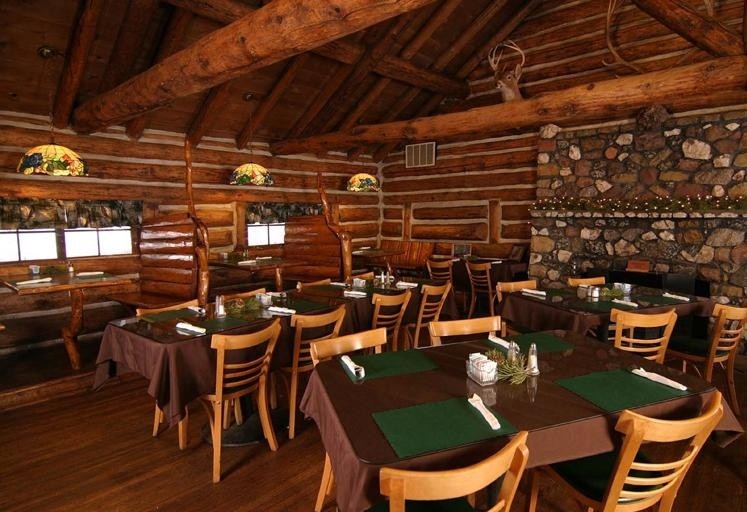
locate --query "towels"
[15,283,52,289]
[76,272,103,276]
[15,277,52,284]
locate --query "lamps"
[230,94,275,186]
[17,43,90,176]
[346,141,381,192]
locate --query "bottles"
[506,340,516,361]
[592,288,600,297]
[587,286,592,296]
[215,295,226,318]
[526,343,540,376]
[386,271,391,284]
[527,377,538,402]
[380,271,384,283]
[67,260,74,271]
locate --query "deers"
[487,39,526,103]
